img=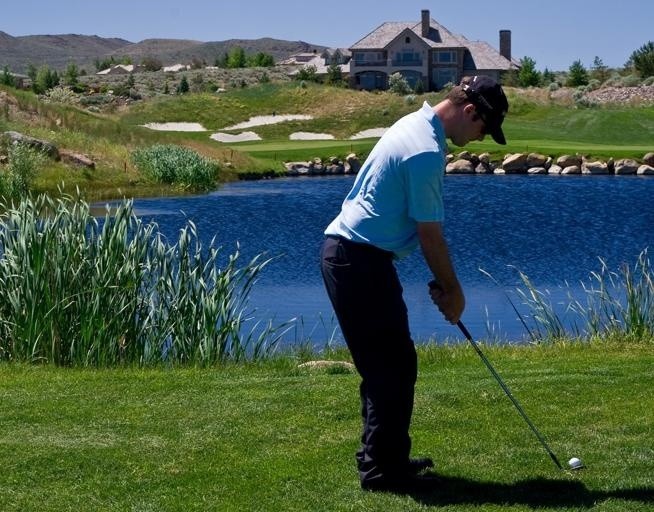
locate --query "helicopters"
[567,456,582,468]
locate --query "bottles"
[461,74,507,146]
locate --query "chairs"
[407,459,434,473]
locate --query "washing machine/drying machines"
[429,279,587,472]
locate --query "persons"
[320,74,509,491]
